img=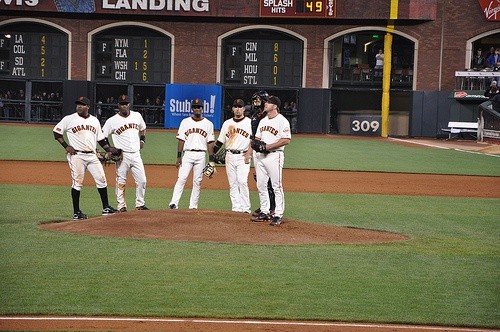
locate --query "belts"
[75,150,93,153]
[226,150,248,154]
[191,150,206,152]
[253,149,282,152]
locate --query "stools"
[335,64,413,83]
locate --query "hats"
[51,93,55,96]
[478,48,482,51]
[492,81,496,85]
[233,99,244,107]
[191,99,204,109]
[75,97,90,106]
[264,95,281,107]
[118,95,131,103]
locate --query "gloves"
[251,139,266,153]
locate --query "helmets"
[252,91,268,109]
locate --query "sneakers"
[72,210,88,220]
[101,206,119,215]
[250,213,270,222]
[269,216,285,225]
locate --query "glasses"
[194,106,202,109]
[234,105,242,108]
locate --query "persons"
[250,91,275,215]
[250,96,292,226]
[52,96,118,221]
[213,97,255,213]
[1,44,500,133]
[101,94,152,210]
[168,98,217,210]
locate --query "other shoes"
[120,207,127,213]
[170,204,176,209]
[136,205,150,210]
[252,208,261,217]
[243,209,251,214]
[155,123,158,127]
[269,210,275,219]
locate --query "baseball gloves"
[202,161,216,180]
[211,149,225,165]
[105,146,124,162]
[97,152,111,161]
[251,138,268,154]
